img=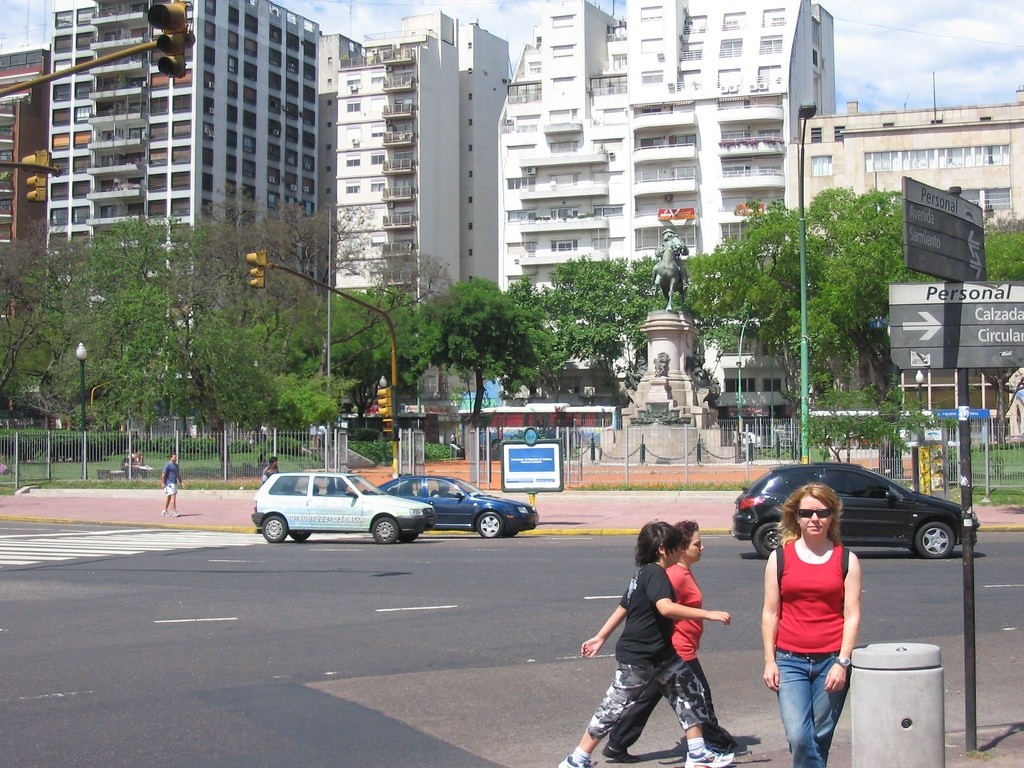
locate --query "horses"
[644,234,689,311]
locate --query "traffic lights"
[246,249,267,289]
[382,419,394,440]
[22,149,52,202]
[377,388,393,417]
[147,0,194,78]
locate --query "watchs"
[836,657,851,666]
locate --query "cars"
[449,430,515,458]
[729,431,763,448]
[376,475,539,539]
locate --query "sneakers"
[559,756,598,768]
[708,738,749,755]
[603,745,639,762]
[684,749,735,768]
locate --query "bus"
[809,410,929,460]
[456,403,623,450]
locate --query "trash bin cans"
[850,641,945,767]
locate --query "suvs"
[728,463,980,560]
[251,472,436,544]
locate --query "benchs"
[97,466,153,480]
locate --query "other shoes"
[173,513,179,517]
[161,512,171,517]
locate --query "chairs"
[430,489,437,497]
[325,482,335,495]
[313,484,319,495]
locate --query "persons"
[120,451,142,479]
[933,451,943,489]
[161,453,183,517]
[761,483,861,768]
[263,457,278,484]
[650,228,689,296]
[438,482,456,498]
[0,463,21,475]
[603,519,747,760]
[558,522,735,768]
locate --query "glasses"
[794,507,831,518]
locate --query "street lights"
[738,317,760,446]
[916,370,924,447]
[76,343,88,480]
[798,103,817,464]
